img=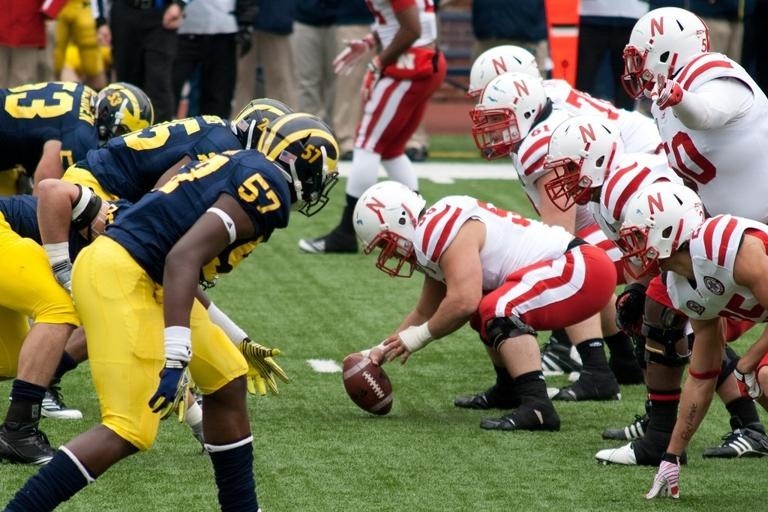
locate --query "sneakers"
[611,363,647,386]
[297,226,361,258]
[478,399,563,432]
[539,342,581,378]
[593,425,690,467]
[551,365,622,403]
[36,390,83,424]
[453,380,522,411]
[0,416,57,466]
[603,401,650,442]
[700,415,767,459]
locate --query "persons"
[469,73,645,401]
[93,0,186,127]
[469,0,551,80]
[290,0,380,160]
[164,0,255,120]
[232,0,299,119]
[595,160,768,458]
[47,0,112,92]
[341,182,617,431]
[0,0,69,89]
[0,83,154,197]
[619,182,768,499]
[0,111,339,512]
[0,179,134,466]
[468,46,667,374]
[8,98,295,419]
[595,7,768,467]
[299,0,448,254]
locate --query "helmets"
[228,95,289,153]
[351,179,429,281]
[255,110,343,220]
[84,78,156,145]
[614,180,706,281]
[619,6,713,103]
[463,44,545,103]
[468,71,545,162]
[599,155,686,237]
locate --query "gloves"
[731,368,764,403]
[332,34,375,77]
[650,71,685,112]
[642,451,684,501]
[361,55,384,101]
[145,355,200,426]
[236,337,295,400]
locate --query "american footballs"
[342,354,394,416]
